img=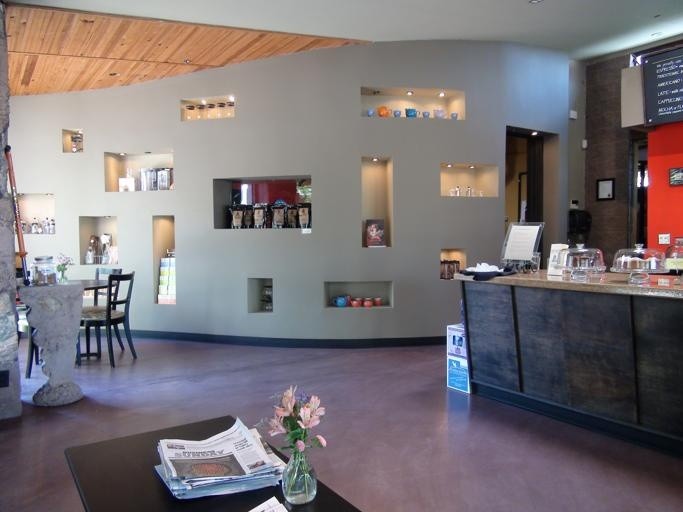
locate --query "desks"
[64,415,360,512]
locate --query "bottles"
[70,134,83,153]
[20,217,55,234]
[448,186,485,198]
[29,255,56,286]
[184,102,235,120]
[126,166,173,192]
[259,285,272,312]
[440,260,460,280]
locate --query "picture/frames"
[596,178,616,201]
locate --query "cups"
[332,295,385,307]
[366,105,458,121]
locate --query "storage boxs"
[447,323,479,394]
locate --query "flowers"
[54,252,74,286]
[267,385,327,505]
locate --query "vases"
[281,455,317,505]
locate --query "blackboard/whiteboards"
[641,44,683,127]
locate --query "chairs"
[15,268,137,379]
